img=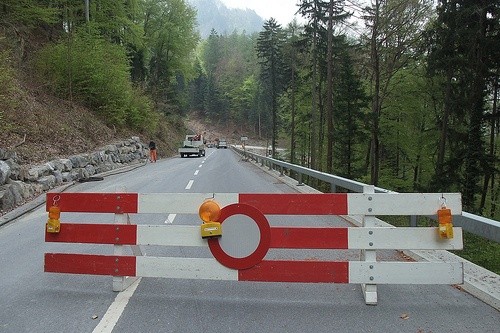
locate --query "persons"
[149,138,157,163]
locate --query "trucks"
[178,135,206,158]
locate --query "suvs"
[217,140,227,149]
